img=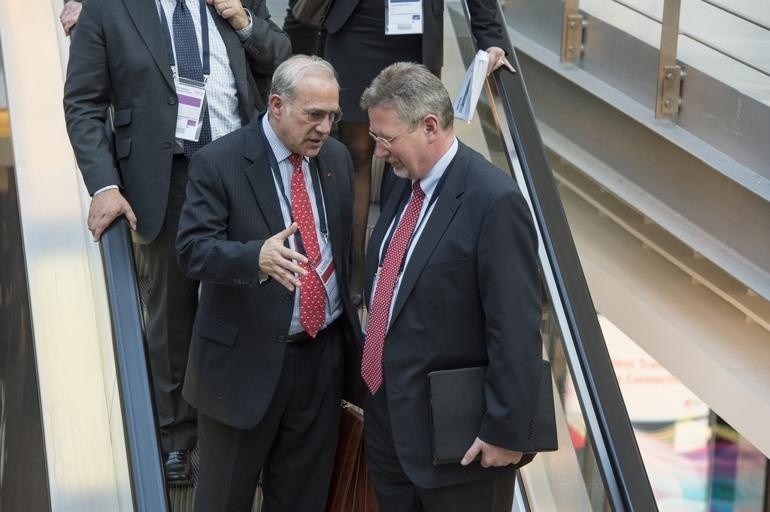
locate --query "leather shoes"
[164,449,194,486]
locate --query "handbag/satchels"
[326,398,381,511]
[292,0,333,32]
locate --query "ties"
[360,178,427,396]
[287,153,328,340]
[172,0,213,161]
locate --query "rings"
[224,2,230,10]
[499,60,505,65]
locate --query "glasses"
[282,91,343,124]
[368,121,414,149]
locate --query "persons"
[358,57,545,512]
[60,0,293,494]
[57,0,83,36]
[283,0,518,310]
[169,50,357,511]
